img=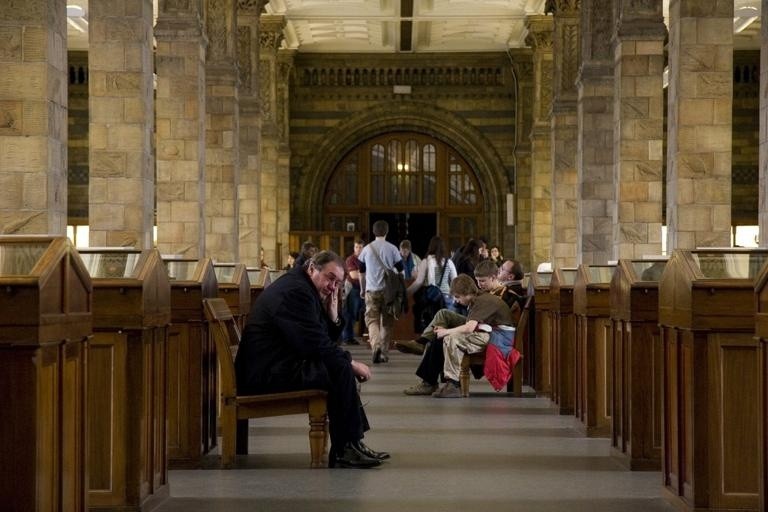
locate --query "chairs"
[459,296,534,398]
[203,297,328,469]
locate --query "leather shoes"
[329,437,391,469]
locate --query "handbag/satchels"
[411,284,441,304]
[384,271,401,305]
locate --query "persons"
[404,258,516,396]
[259,220,525,366]
[433,272,516,398]
[234,247,392,468]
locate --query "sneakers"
[371,343,388,362]
[405,380,461,398]
[392,341,425,355]
